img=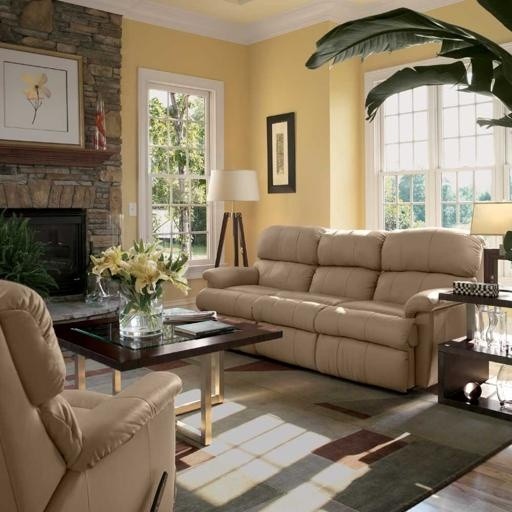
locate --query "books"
[174,320,234,336]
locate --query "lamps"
[470,200,512,249]
[207,169,259,268]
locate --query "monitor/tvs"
[0,207,88,296]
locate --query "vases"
[118,284,163,350]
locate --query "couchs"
[0,277,183,512]
[196,223,485,395]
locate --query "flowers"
[87,236,190,329]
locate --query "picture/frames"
[266,112,296,194]
[0,42,86,150]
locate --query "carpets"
[59,339,512,511]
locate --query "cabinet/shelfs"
[436,247,512,421]
